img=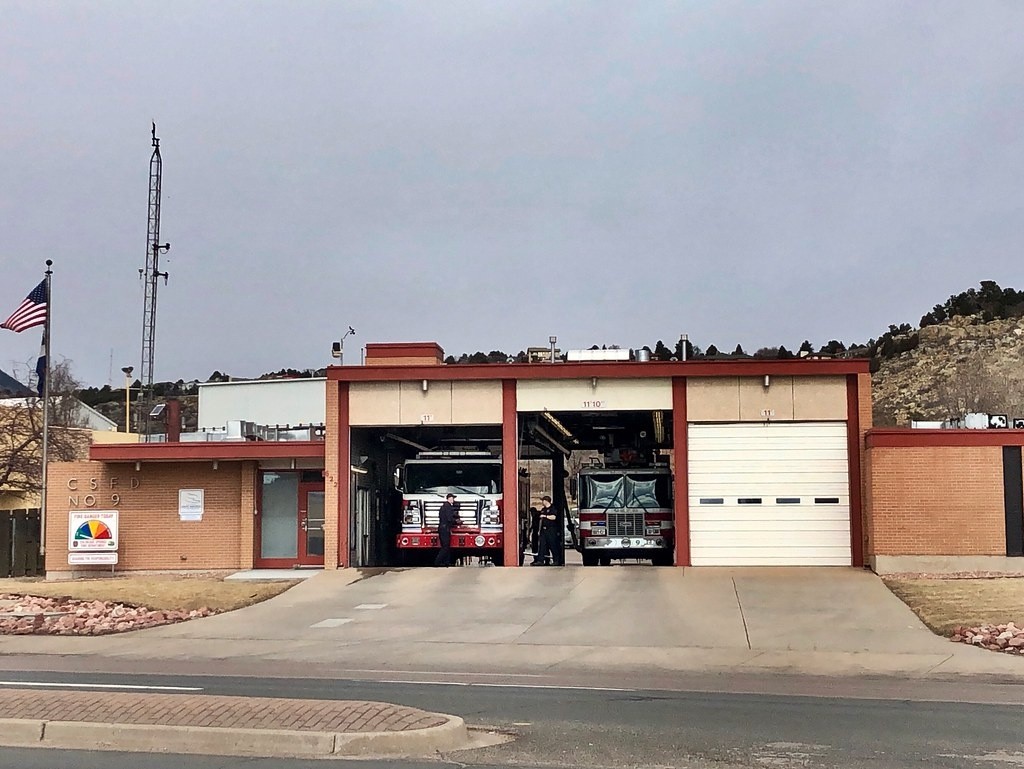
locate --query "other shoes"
[435,560,453,566]
[551,560,565,566]
[530,560,545,565]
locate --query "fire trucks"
[567,451,676,567]
[392,448,531,567]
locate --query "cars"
[564,507,580,549]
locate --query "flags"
[0,275,48,333]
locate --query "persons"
[432,493,464,567]
[529,496,561,566]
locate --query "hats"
[540,496,552,503]
[446,494,457,499]
